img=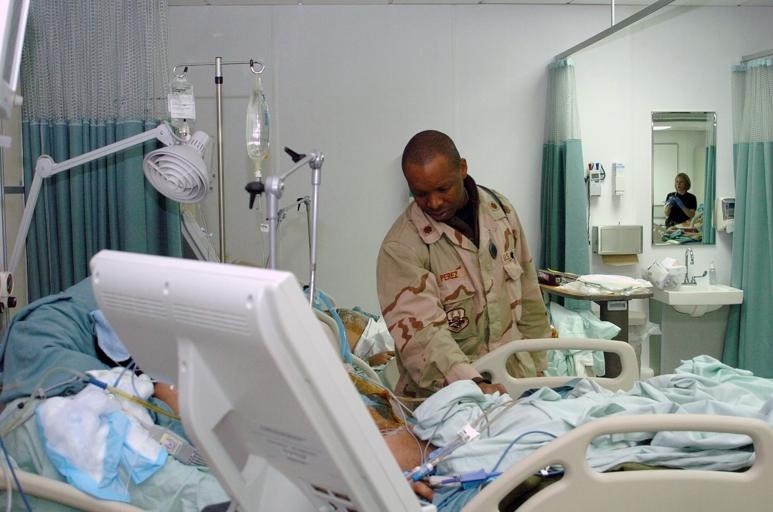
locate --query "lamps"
[4,124,213,308]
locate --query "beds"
[3,275,773,512]
[325,292,617,389]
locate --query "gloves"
[667,195,674,207]
[674,197,684,208]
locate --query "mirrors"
[651,110,717,246]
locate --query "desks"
[538,282,653,379]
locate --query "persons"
[376,130,553,395]
[149,376,443,484]
[663,173,697,228]
[319,305,396,369]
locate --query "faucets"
[685,247,694,283]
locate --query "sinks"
[670,286,724,291]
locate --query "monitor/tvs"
[89,249,422,512]
